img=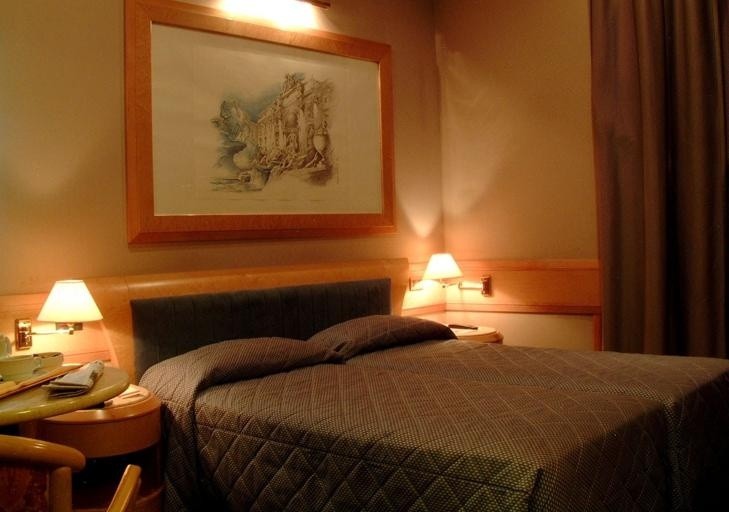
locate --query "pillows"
[138,337,327,512]
[308,314,458,365]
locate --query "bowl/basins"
[0,352,66,383]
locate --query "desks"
[0,367,129,438]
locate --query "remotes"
[448,321,478,331]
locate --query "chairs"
[0,435,142,512]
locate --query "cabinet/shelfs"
[446,324,504,344]
[41,383,165,512]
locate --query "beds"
[87,257,729,512]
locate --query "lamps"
[410,253,464,292]
[15,279,104,350]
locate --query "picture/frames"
[125,0,398,245]
